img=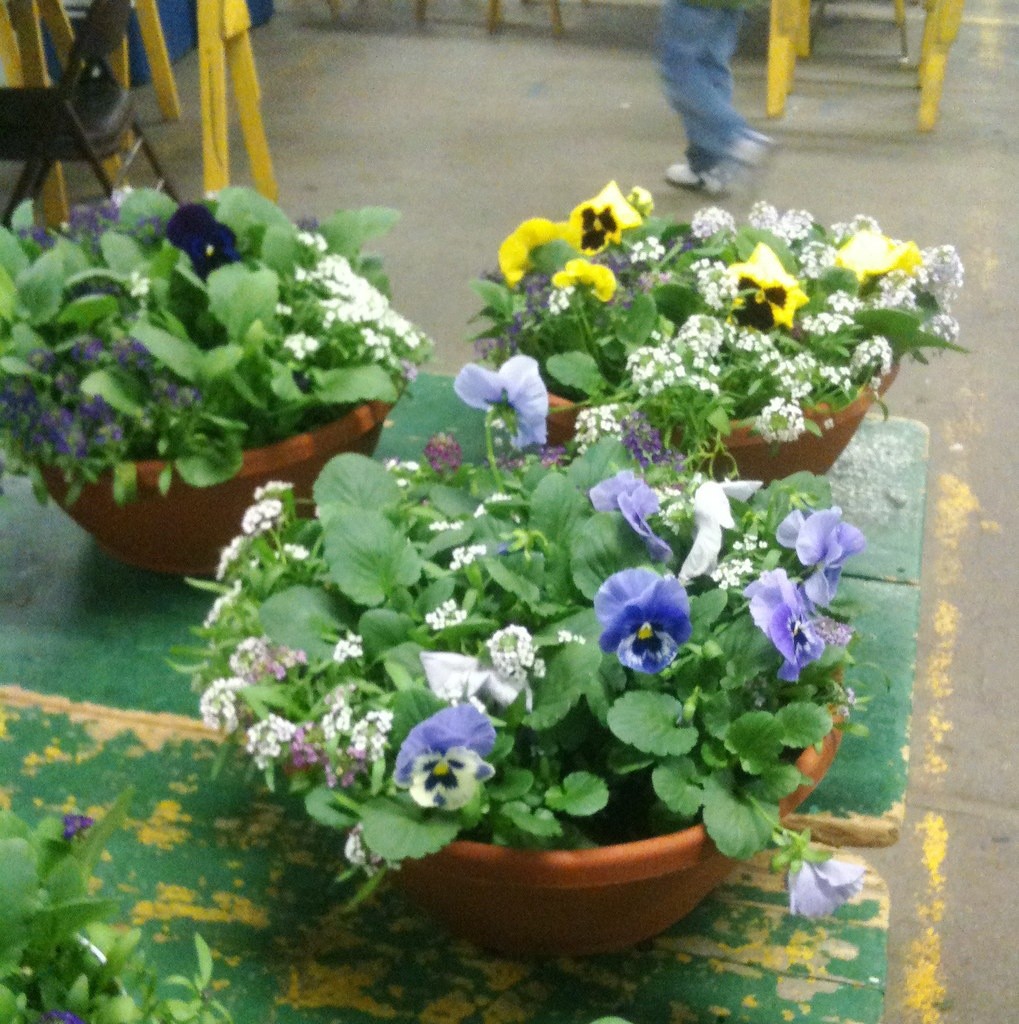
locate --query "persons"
[651,0,785,199]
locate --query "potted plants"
[40,399,387,572]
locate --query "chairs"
[0,0,185,217]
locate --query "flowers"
[1,182,439,506]
[162,355,866,922]
[0,785,237,1024]
[475,182,974,449]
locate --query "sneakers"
[665,163,733,198]
[730,126,781,204]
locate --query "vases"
[395,726,843,957]
[542,357,899,487]
